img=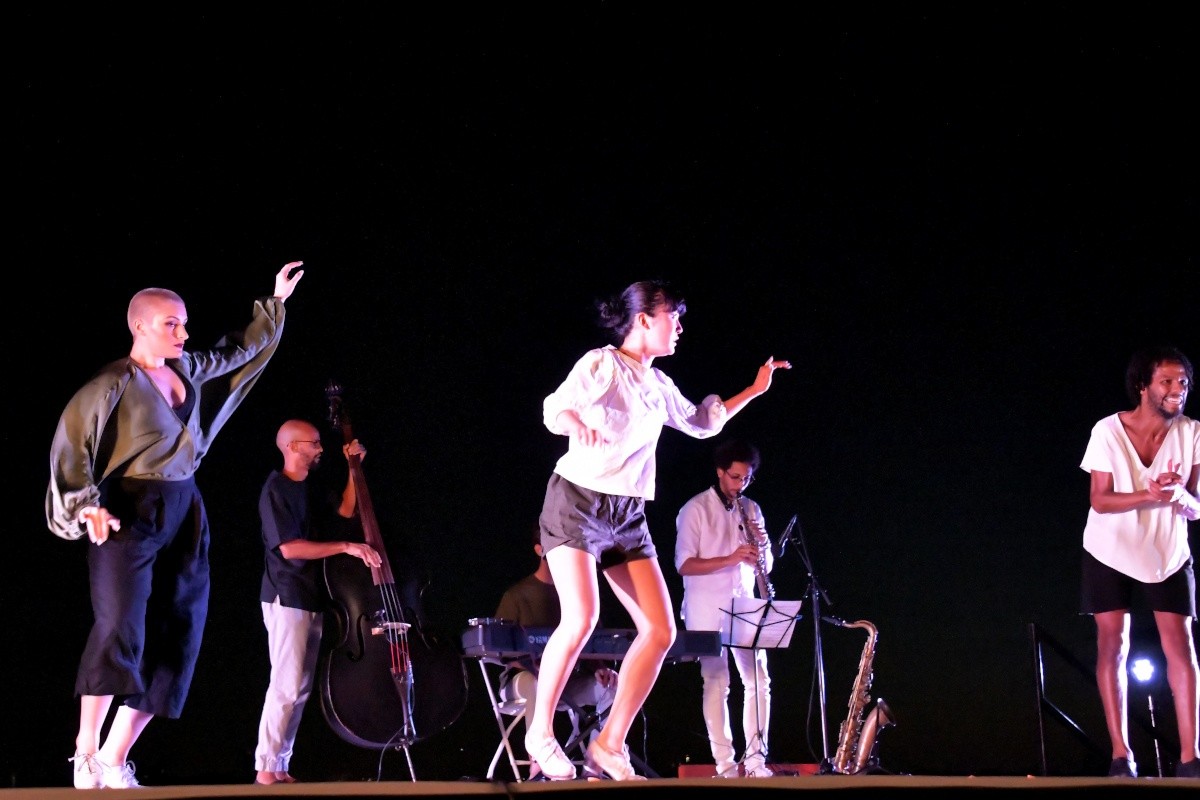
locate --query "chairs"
[479,660,586,782]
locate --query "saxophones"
[817,613,899,776]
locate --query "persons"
[675,444,774,779]
[46,264,303,794]
[494,530,617,785]
[525,282,792,784]
[254,421,382,785]
[1078,348,1199,778]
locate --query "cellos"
[297,377,474,782]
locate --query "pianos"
[462,616,723,782]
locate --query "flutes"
[734,488,778,600]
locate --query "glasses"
[285,439,320,447]
[724,470,755,485]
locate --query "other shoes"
[68,748,106,789]
[1176,759,1200,776]
[748,766,773,777]
[90,753,144,789]
[1109,758,1137,779]
[524,729,577,780]
[725,769,740,779]
[586,738,649,782]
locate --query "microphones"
[771,514,797,557]
